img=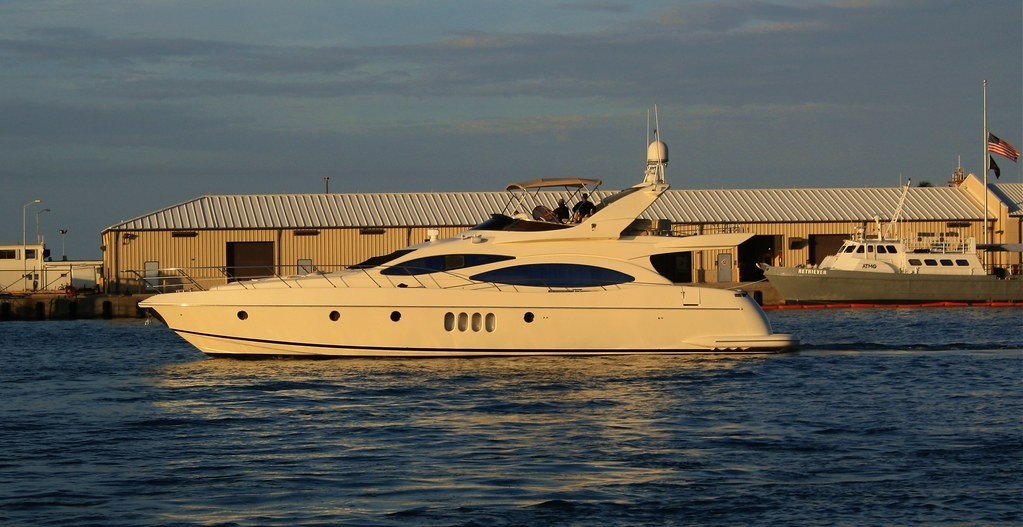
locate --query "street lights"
[36,208,52,243]
[23,198,42,294]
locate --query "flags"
[988,131,1020,162]
[988,154,1000,179]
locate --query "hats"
[581,193,588,198]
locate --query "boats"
[755,172,1023,311]
[132,101,807,357]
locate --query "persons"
[570,193,595,223]
[539,199,569,223]
